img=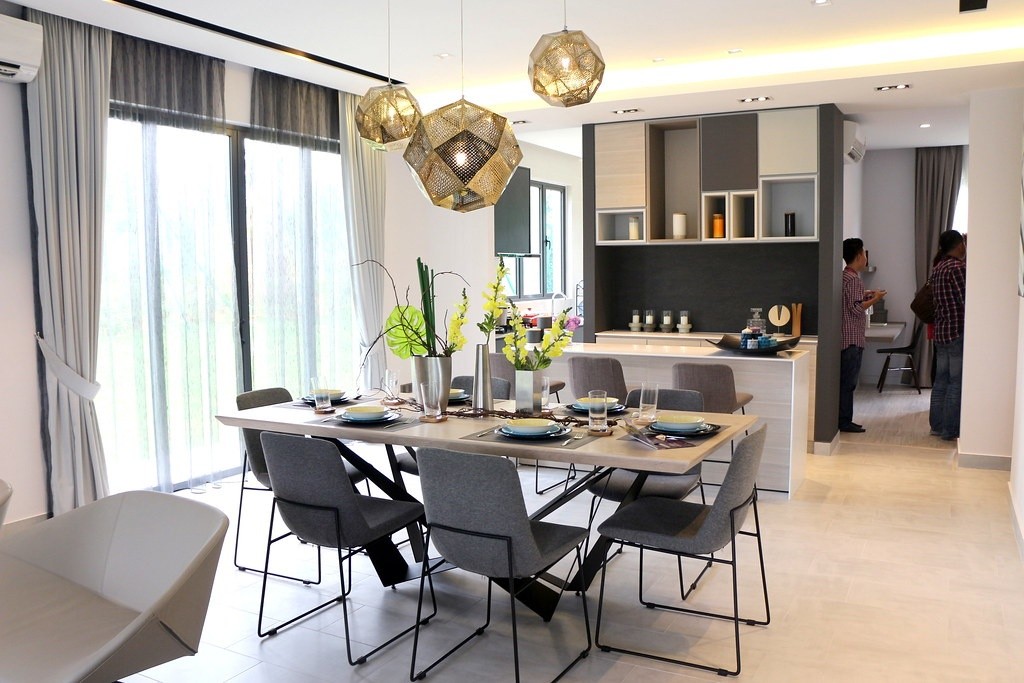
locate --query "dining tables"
[212,388,751,597]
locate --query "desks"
[864,320,906,343]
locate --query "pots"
[523,307,540,327]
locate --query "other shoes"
[840,424,866,432]
[941,428,960,440]
[929,425,945,435]
[850,421,862,428]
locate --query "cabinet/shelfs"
[592,114,700,247]
[701,106,825,246]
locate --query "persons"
[927,229,967,441]
[838,238,888,433]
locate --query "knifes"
[477,424,500,436]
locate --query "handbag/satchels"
[910,279,936,324]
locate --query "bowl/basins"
[506,418,555,433]
[449,388,465,396]
[345,405,388,417]
[310,389,344,399]
[657,413,704,429]
[577,396,619,408]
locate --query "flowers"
[475,252,506,344]
[352,253,472,380]
[500,299,575,369]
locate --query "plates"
[494,427,572,440]
[335,413,401,425]
[649,423,719,437]
[446,394,469,403]
[566,402,624,413]
[300,395,348,405]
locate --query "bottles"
[745,307,766,332]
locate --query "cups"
[662,310,671,325]
[632,310,639,323]
[311,375,331,410]
[645,309,653,324]
[589,388,607,431]
[628,217,639,240]
[420,381,442,419]
[739,333,778,350]
[680,310,688,324]
[711,213,724,237]
[638,381,659,420]
[385,368,401,400]
[785,211,796,237]
[542,377,550,405]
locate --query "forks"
[562,432,584,446]
[384,416,417,429]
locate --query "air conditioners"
[0,14,44,85]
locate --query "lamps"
[527,0,608,109]
[400,0,522,215]
[354,0,424,151]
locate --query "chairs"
[1,488,231,683]
[586,384,715,603]
[566,354,628,409]
[393,374,510,476]
[673,361,762,502]
[489,351,577,494]
[399,447,597,683]
[876,318,925,395]
[233,388,369,584]
[593,426,773,677]
[252,432,436,667]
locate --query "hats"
[938,230,962,252]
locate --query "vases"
[514,369,542,414]
[473,344,495,411]
[409,356,451,415]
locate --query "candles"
[646,310,654,323]
[679,310,688,325]
[632,310,639,323]
[663,310,672,324]
